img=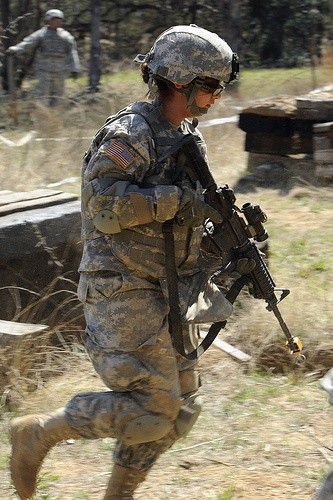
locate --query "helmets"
[45,9,64,19]
[133,24,239,89]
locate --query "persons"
[6,9,82,107]
[9,25,266,500]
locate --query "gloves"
[176,188,223,226]
[224,253,256,278]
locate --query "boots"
[9,408,82,500]
[104,464,147,500]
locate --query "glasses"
[194,78,226,96]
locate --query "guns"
[156,134,297,356]
